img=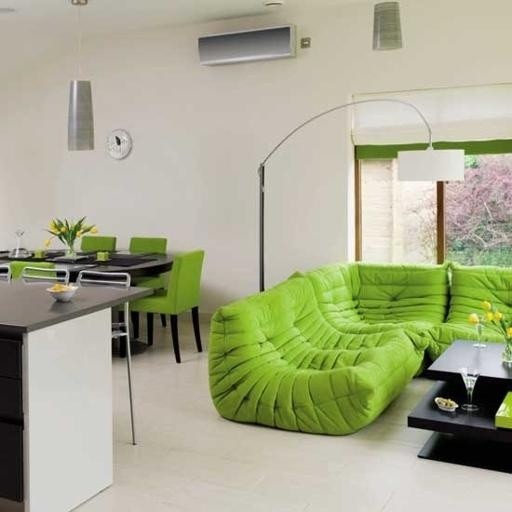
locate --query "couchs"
[209,263,512,436]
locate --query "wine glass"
[472,324,486,348]
[458,369,480,412]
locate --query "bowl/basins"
[47,287,79,302]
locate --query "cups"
[35,251,45,258]
[97,252,109,261]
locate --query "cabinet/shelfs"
[0,280,152,511]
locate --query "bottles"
[8,225,32,259]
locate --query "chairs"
[0,228,205,364]
[76,270,138,447]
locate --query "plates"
[94,259,112,263]
[32,257,48,259]
[435,396,458,412]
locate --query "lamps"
[257,96,467,292]
[368,0,404,51]
[65,0,96,150]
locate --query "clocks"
[105,128,133,161]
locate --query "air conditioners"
[198,22,295,65]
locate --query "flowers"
[41,216,99,247]
